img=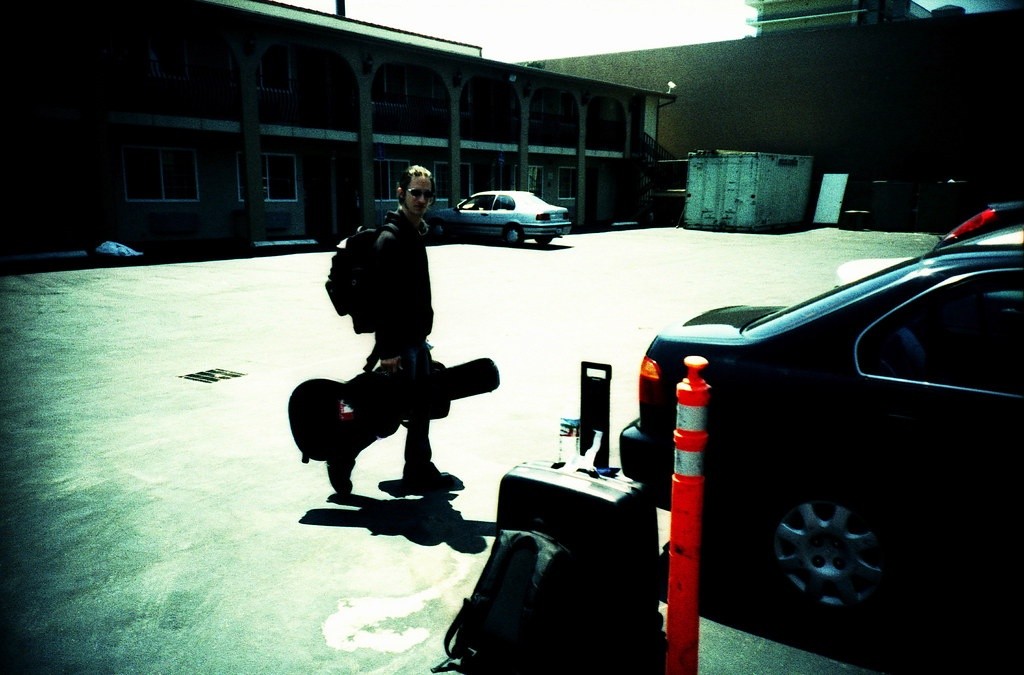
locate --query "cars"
[619,200,1024,617]
[422,189,573,247]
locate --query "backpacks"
[444,528,594,675]
[326,223,400,334]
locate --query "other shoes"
[328,460,352,497]
[401,464,464,490]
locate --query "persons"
[327,166,466,497]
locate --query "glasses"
[406,189,433,198]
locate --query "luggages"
[497,460,669,674]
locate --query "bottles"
[560,403,580,463]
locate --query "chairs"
[482,201,490,209]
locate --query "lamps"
[508,74,518,83]
